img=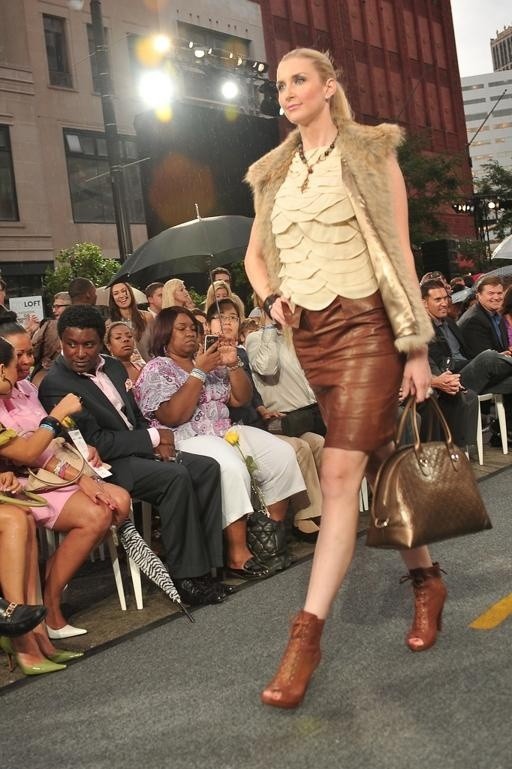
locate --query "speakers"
[422,239,460,287]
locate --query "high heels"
[0,635,67,675]
[263,609,326,709]
[45,648,85,663]
[398,560,448,653]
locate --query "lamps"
[172,36,272,82]
[450,193,512,215]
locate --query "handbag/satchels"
[246,484,292,572]
[257,401,327,437]
[366,388,494,552]
[17,429,112,493]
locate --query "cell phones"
[205,335,218,355]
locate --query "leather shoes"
[0,597,47,638]
[46,621,88,639]
[227,556,272,581]
[493,432,512,447]
[291,521,319,544]
[176,578,228,606]
[203,574,236,595]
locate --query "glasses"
[210,314,241,325]
[431,271,443,277]
[52,304,69,308]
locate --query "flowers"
[222,428,266,498]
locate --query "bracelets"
[55,456,69,481]
[261,296,279,318]
[39,413,63,437]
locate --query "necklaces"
[294,133,343,191]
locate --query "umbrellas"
[106,202,252,343]
[490,233,512,264]
[449,265,511,301]
[115,518,196,623]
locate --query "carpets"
[0,425,512,696]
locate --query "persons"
[0,599,54,637]
[242,46,448,714]
[3,313,131,639]
[0,274,511,604]
[3,337,84,681]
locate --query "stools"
[39,493,155,614]
[465,392,509,466]
